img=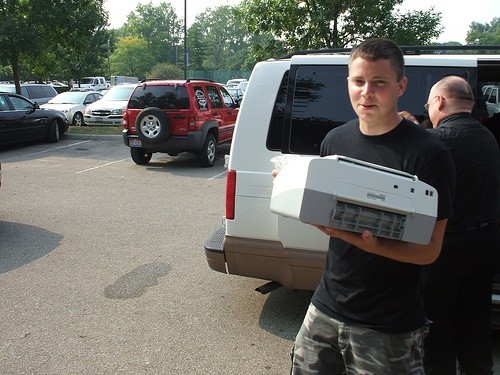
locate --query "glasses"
[424,95,445,110]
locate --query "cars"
[38,92,103,126]
[82,84,138,127]
[0,91,70,148]
[0,77,110,104]
[224,78,248,107]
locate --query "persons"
[397,76,500,375]
[272,38,458,375]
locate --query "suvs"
[122,78,239,168]
[203,43,500,296]
